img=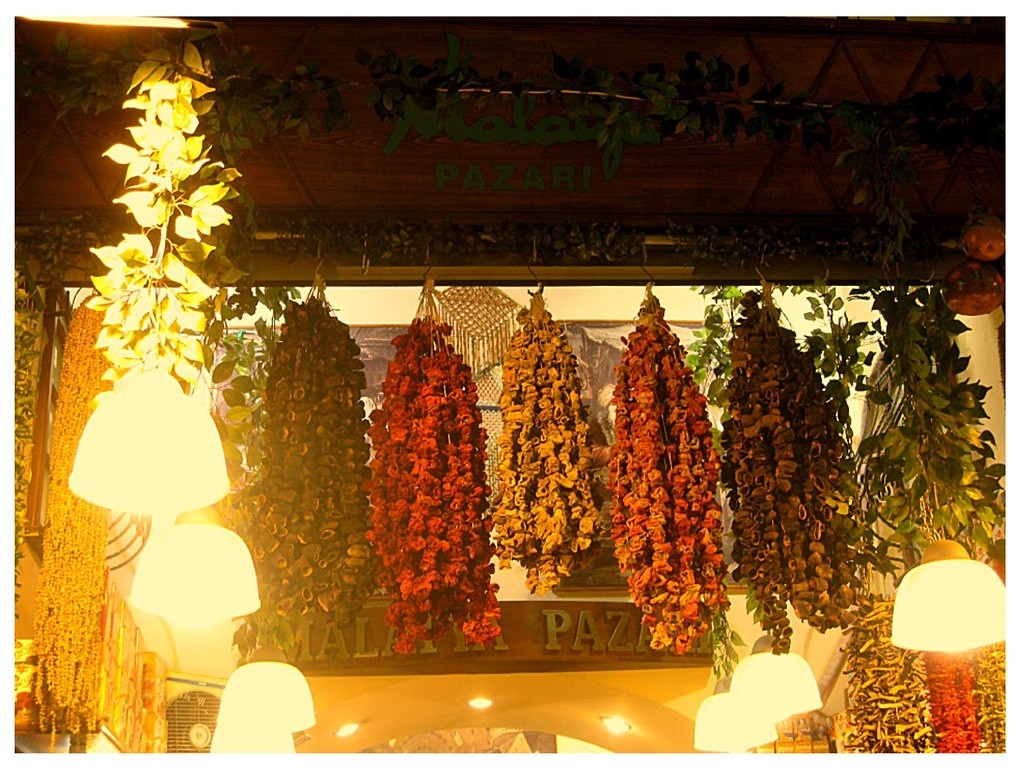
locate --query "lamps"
[695,600,824,753]
[66,308,318,753]
[893,485,1006,653]
[643,234,692,266]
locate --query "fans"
[164,668,230,753]
